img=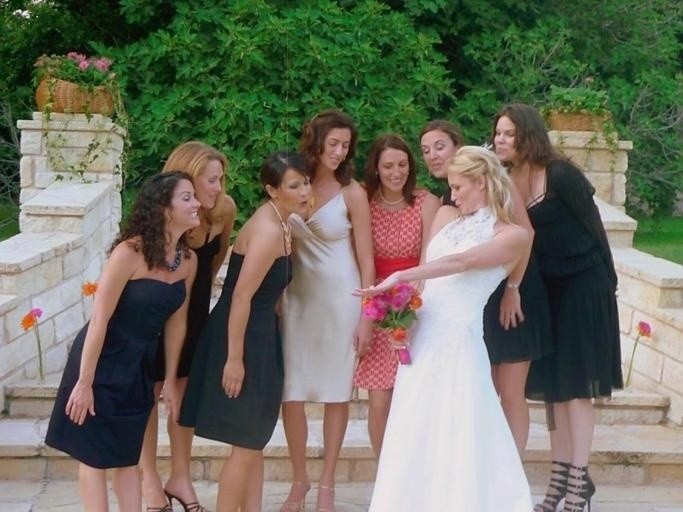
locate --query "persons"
[45,170,201,511]
[494,105,625,512]
[349,145,530,512]
[277,111,374,511]
[420,121,536,459]
[178,151,314,512]
[139,142,237,511]
[359,137,440,461]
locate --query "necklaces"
[309,177,336,209]
[378,186,406,206]
[269,200,293,259]
[160,246,182,271]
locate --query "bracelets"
[502,282,520,291]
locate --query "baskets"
[34,74,122,117]
[546,107,613,131]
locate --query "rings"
[369,285,374,289]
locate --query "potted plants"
[539,79,619,204]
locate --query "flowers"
[362,284,422,365]
[31,52,132,188]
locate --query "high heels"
[565,463,594,512]
[147,498,172,512]
[279,477,310,512]
[534,460,569,512]
[163,489,207,512]
[314,482,338,512]
